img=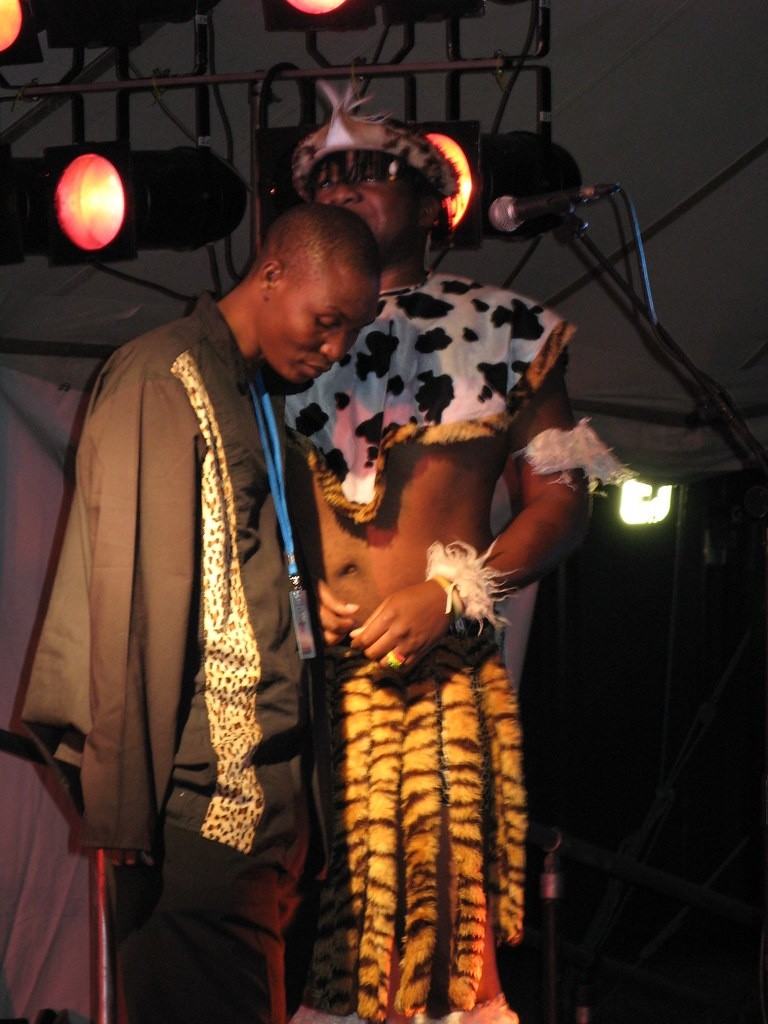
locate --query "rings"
[387,649,406,670]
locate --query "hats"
[291,77,458,206]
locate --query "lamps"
[43,139,247,269]
[0,0,76,66]
[261,0,381,33]
[410,118,583,251]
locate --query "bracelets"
[433,574,465,622]
[424,534,520,636]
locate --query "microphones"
[488,183,621,233]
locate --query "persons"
[293,76,636,1024]
[21,198,381,1024]
